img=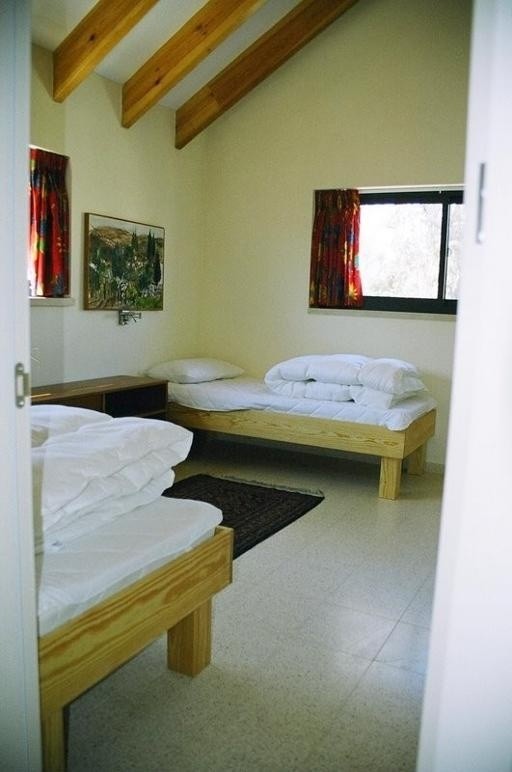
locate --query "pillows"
[143,357,246,383]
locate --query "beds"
[166,371,438,504]
[34,497,237,772]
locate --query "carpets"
[162,473,324,559]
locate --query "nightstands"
[30,375,170,423]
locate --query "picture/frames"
[82,212,166,313]
